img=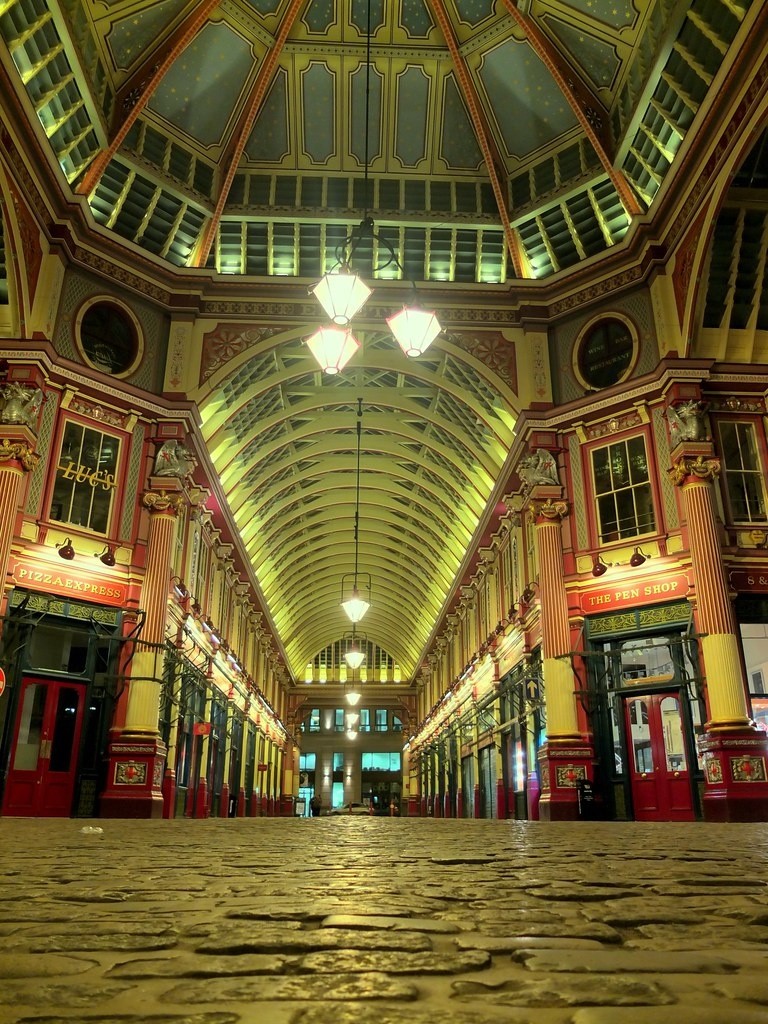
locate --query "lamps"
[592,556,613,577]
[339,397,372,625]
[94,546,115,567]
[341,625,370,742]
[56,537,75,560]
[430,582,540,719]
[169,574,251,680]
[302,0,443,381]
[630,546,651,568]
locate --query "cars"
[329,801,375,815]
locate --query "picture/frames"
[751,670,766,693]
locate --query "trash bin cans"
[228,793,238,818]
[576,779,595,820]
[70,768,100,818]
